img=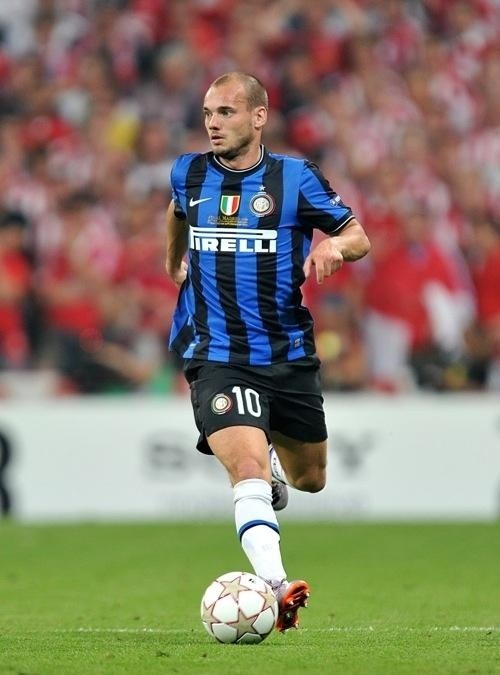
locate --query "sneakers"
[269,447,288,511]
[271,577,309,633]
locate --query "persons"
[2,0,500,390]
[164,71,372,631]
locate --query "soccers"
[200,571,279,644]
